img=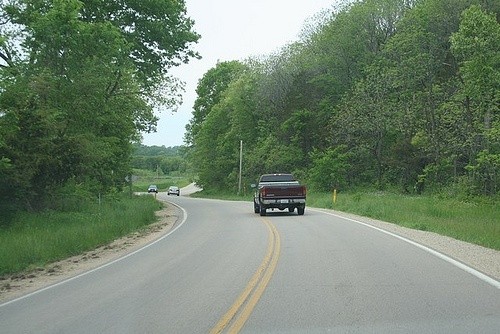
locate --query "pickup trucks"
[251,173,306,216]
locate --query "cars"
[168,185,179,196]
[148,184,158,193]
